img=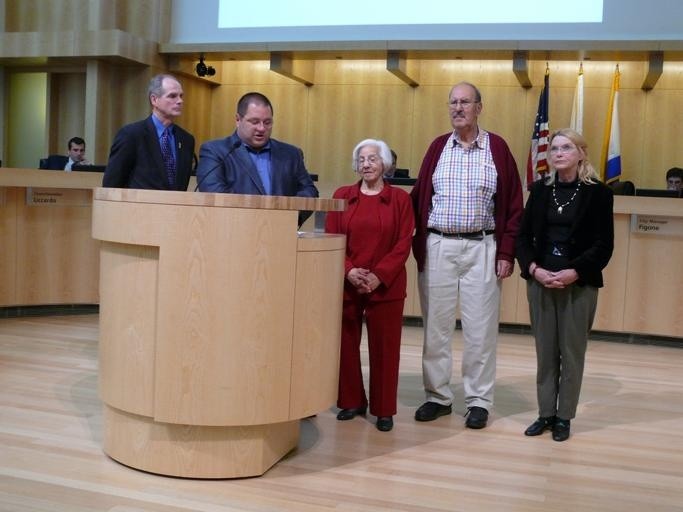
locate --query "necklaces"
[552,183,582,214]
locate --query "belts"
[432,227,495,237]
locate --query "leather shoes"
[377,415,393,431]
[337,404,366,419]
[464,406,488,428]
[525,415,555,436]
[415,401,452,421]
[552,419,570,441]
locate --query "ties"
[161,128,176,188]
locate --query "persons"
[665,166,682,191]
[40,136,90,171]
[383,149,408,178]
[515,128,613,441]
[196,92,318,229]
[102,73,194,190]
[409,82,523,429]
[327,138,416,432]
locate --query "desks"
[0,168,683,342]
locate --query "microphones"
[193,139,242,192]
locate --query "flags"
[571,68,586,133]
[526,70,551,192]
[600,72,622,184]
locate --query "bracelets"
[532,266,539,277]
